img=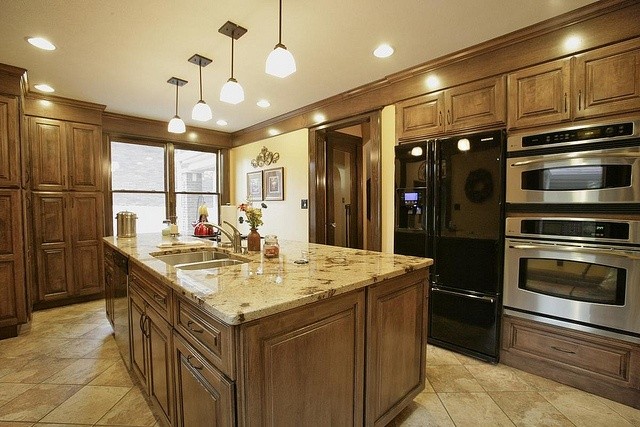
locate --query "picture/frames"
[263,167,284,201]
[246,171,262,202]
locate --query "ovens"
[502,115,640,345]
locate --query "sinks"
[172,258,253,270]
[149,250,230,266]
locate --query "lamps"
[187,54,212,123]
[264,0,297,79]
[167,77,187,133]
[217,21,247,105]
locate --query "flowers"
[237,202,267,228]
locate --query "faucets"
[202,223,234,246]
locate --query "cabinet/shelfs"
[174,291,238,427]
[394,73,507,141]
[366,267,428,426]
[31,192,106,310]
[1,188,28,341]
[24,99,107,192]
[1,61,29,189]
[507,36,640,130]
[128,258,173,427]
[498,311,640,410]
[103,241,115,335]
[240,289,364,426]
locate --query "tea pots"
[191,222,213,236]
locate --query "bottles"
[162,217,171,236]
[262,234,279,257]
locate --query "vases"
[248,228,260,251]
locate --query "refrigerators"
[393,128,507,365]
